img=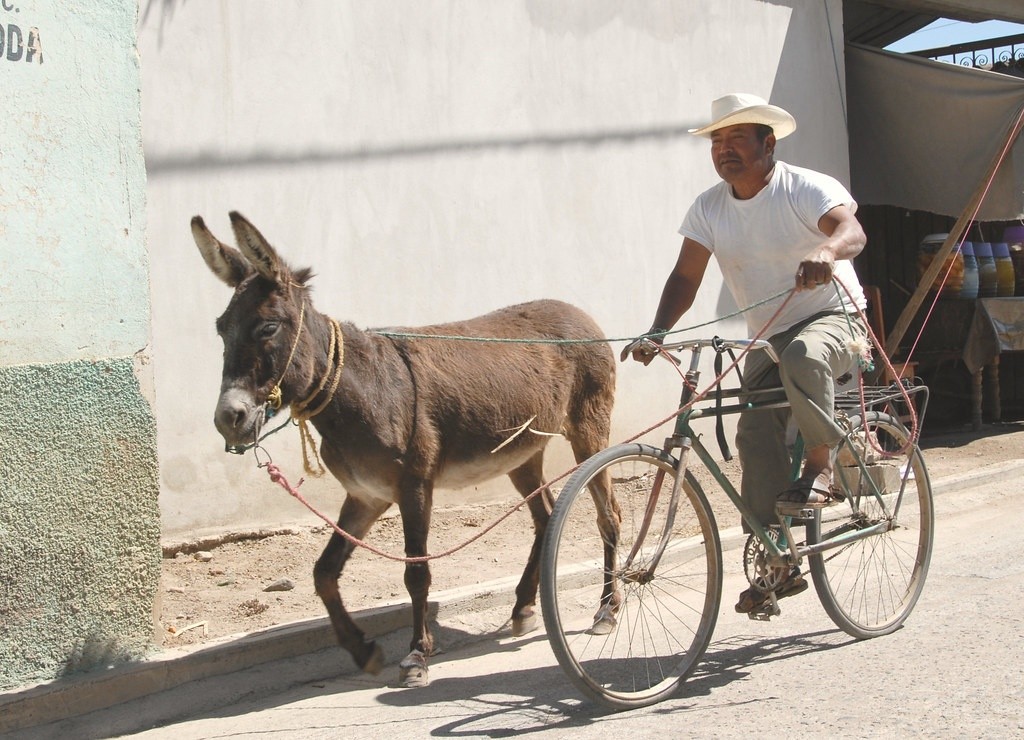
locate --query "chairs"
[864,285,921,453]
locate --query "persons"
[618,94,868,613]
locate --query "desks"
[908,296,1024,432]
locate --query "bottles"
[918,226,1023,299]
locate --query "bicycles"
[540,337,935,706]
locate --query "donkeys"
[190,211,621,688]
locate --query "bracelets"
[648,326,667,341]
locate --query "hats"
[688,93,796,141]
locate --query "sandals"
[775,478,839,509]
[735,565,808,613]
